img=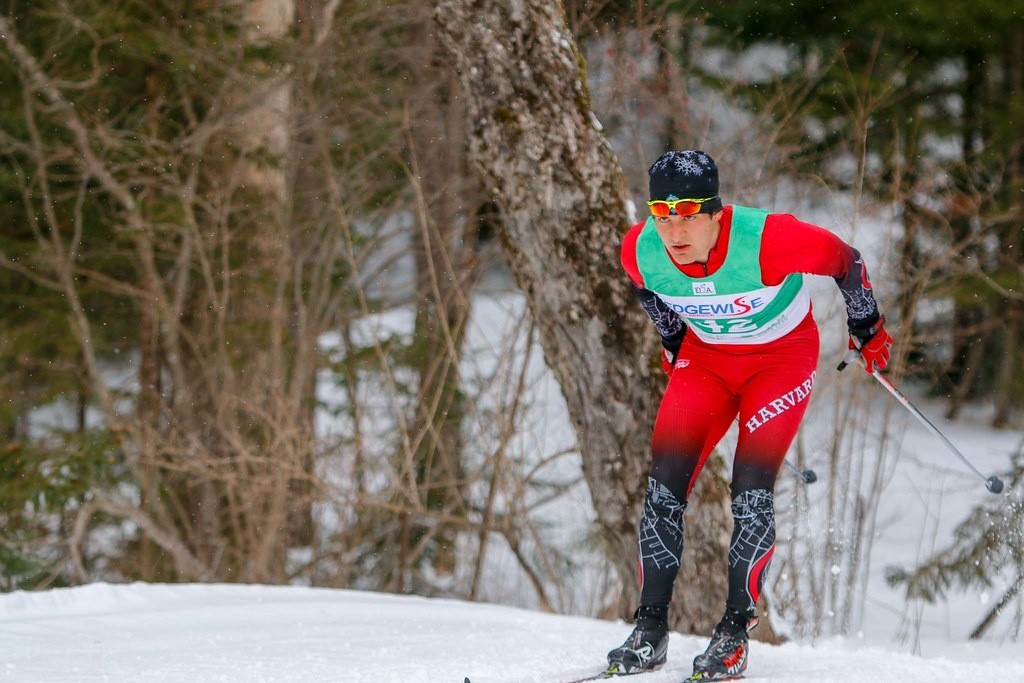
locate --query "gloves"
[848,315,895,375]
[659,348,674,379]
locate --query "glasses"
[645,195,718,216]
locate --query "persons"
[607,149,895,679]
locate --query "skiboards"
[463,671,749,683]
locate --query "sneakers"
[684,610,760,680]
[606,606,669,675]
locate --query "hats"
[648,150,719,201]
